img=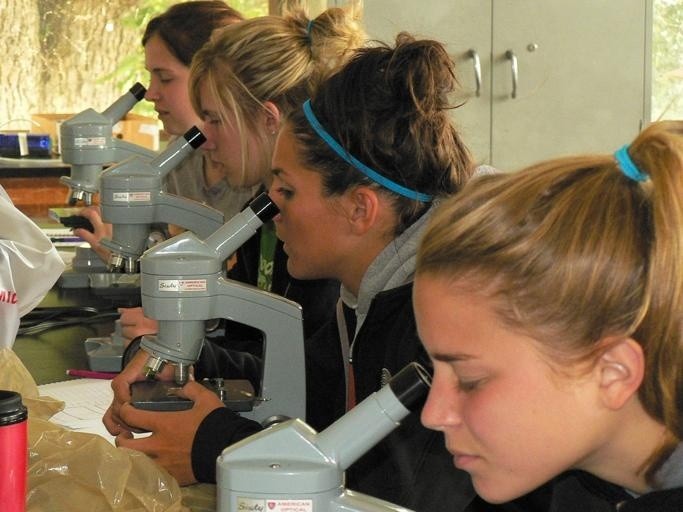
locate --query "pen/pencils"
[66,369,120,379]
[51,238,87,242]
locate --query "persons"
[101,37,460,483]
[412,102,683,511]
[117,0,371,349]
[75,0,252,265]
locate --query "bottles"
[0,390,28,512]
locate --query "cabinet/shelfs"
[265,0,654,181]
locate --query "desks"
[0,222,217,511]
[0,153,103,206]
